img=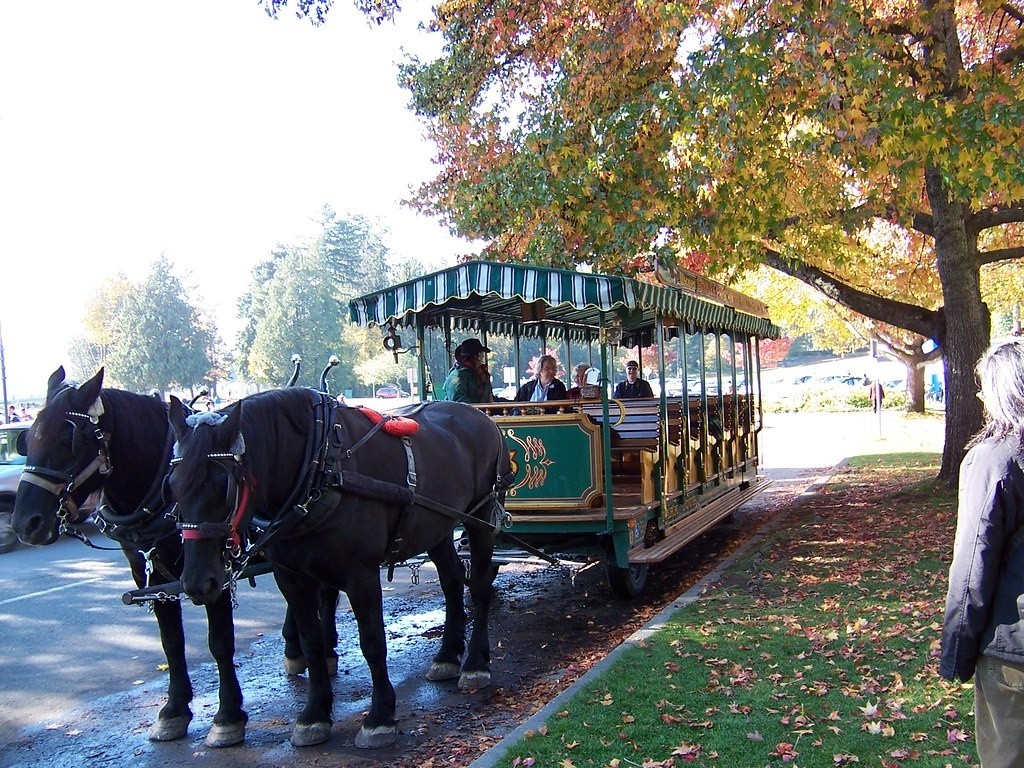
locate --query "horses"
[167,388,512,750]
[9,365,339,750]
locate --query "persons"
[612,361,654,400]
[510,355,567,416]
[337,392,346,404]
[869,380,885,414]
[937,336,1024,768]
[927,384,943,402]
[442,338,512,416]
[566,363,603,400]
[9,404,34,422]
[862,373,870,386]
[727,381,733,394]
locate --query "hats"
[626,361,638,368]
[455,338,490,363]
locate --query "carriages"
[7,261,780,751]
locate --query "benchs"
[572,392,755,505]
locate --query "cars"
[377,386,411,399]
[0,422,103,555]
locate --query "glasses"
[628,368,637,370]
